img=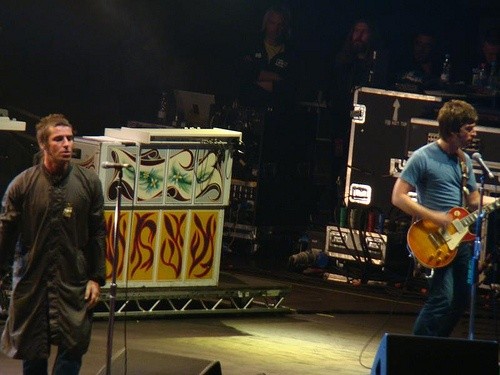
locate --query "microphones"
[101,161,131,168]
[472,153,494,180]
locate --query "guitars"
[406,197,500,270]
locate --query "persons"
[229,2,500,206]
[0,115,108,375]
[392,99,500,336]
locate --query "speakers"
[370,333,500,375]
[95,345,223,375]
